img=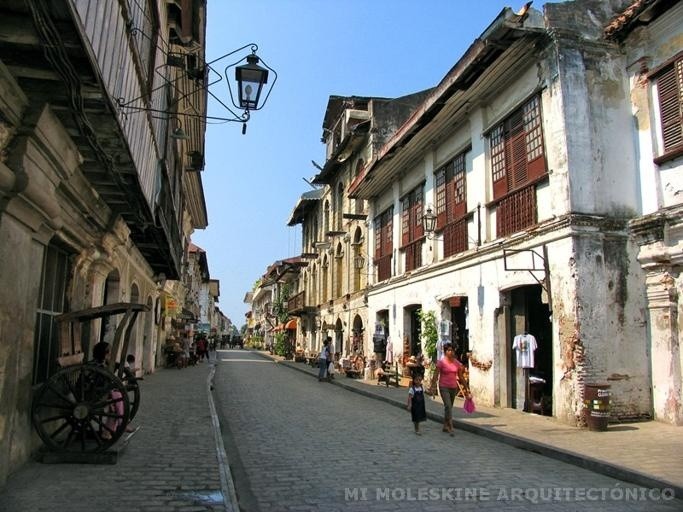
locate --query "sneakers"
[125,427,136,432]
[319,375,335,382]
[442,423,455,432]
[416,431,421,436]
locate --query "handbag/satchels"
[465,398,475,414]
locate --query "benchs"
[377,361,399,389]
[59,353,143,452]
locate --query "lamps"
[422,206,445,242]
[349,252,376,274]
[117,24,274,134]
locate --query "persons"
[89,342,137,444]
[121,354,141,379]
[318,340,332,381]
[427,342,471,436]
[325,335,335,379]
[182,332,211,366]
[405,371,436,436]
